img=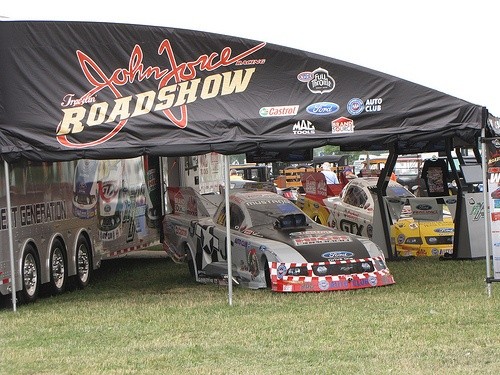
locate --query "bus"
[0,153,234,302]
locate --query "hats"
[322,162,330,170]
[343,168,352,173]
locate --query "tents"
[0,20,499,315]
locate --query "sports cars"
[162,190,396,292]
[296,176,454,258]
